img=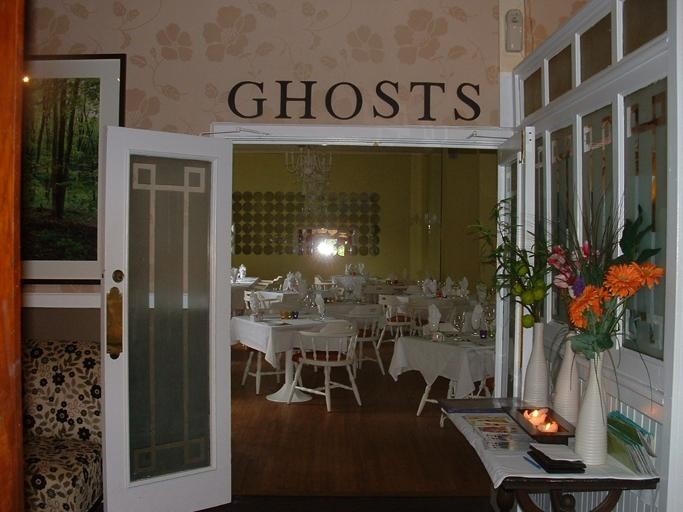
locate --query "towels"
[286,272,295,291]
[240,263,247,280]
[344,263,352,275]
[295,272,302,291]
[354,263,364,275]
[231,266,238,282]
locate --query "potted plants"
[464,196,558,408]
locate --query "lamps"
[284,145,331,217]
[421,208,437,236]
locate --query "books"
[461,415,530,454]
[526,409,661,477]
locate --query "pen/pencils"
[523,456,541,469]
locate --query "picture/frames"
[21,54,123,285]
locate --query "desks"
[441,409,659,512]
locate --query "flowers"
[580,190,665,428]
[526,191,595,390]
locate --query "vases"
[552,331,581,424]
[575,350,607,464]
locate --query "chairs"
[21,341,102,512]
[230,277,496,429]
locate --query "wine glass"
[471,319,480,337]
[317,304,327,321]
[451,312,466,342]
